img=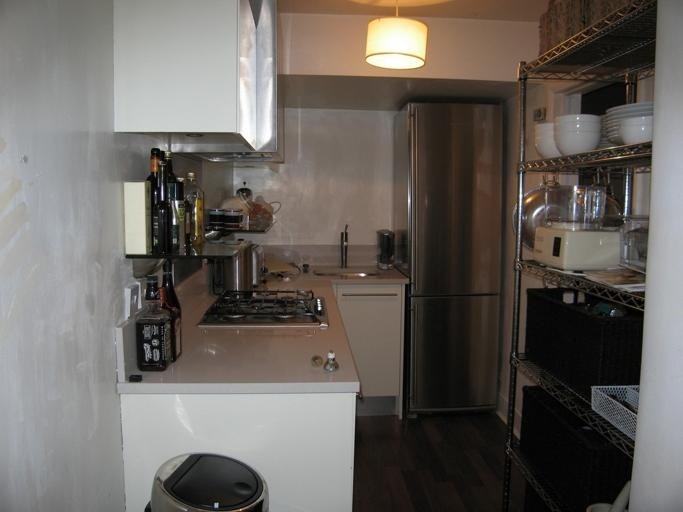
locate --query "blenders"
[533,184,623,272]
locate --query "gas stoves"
[197,290,327,326]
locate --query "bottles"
[151,163,175,253]
[161,260,181,359]
[135,274,172,372]
[164,153,184,252]
[175,178,191,255]
[183,173,204,245]
[157,286,177,363]
[145,149,161,207]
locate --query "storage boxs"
[526,288,645,403]
[519,384,634,511]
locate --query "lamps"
[366,6,429,71]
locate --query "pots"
[211,239,253,293]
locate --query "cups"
[224,209,246,230]
[210,208,224,230]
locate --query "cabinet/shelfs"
[114,0,286,163]
[506,1,658,511]
[332,284,406,417]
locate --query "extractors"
[113,0,285,164]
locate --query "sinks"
[313,266,381,278]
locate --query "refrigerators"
[391,102,504,420]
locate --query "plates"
[605,103,656,146]
[595,114,616,150]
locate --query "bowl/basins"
[512,186,570,250]
[617,114,655,146]
[554,113,601,155]
[532,122,563,157]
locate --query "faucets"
[339,223,351,266]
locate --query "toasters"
[249,243,267,289]
